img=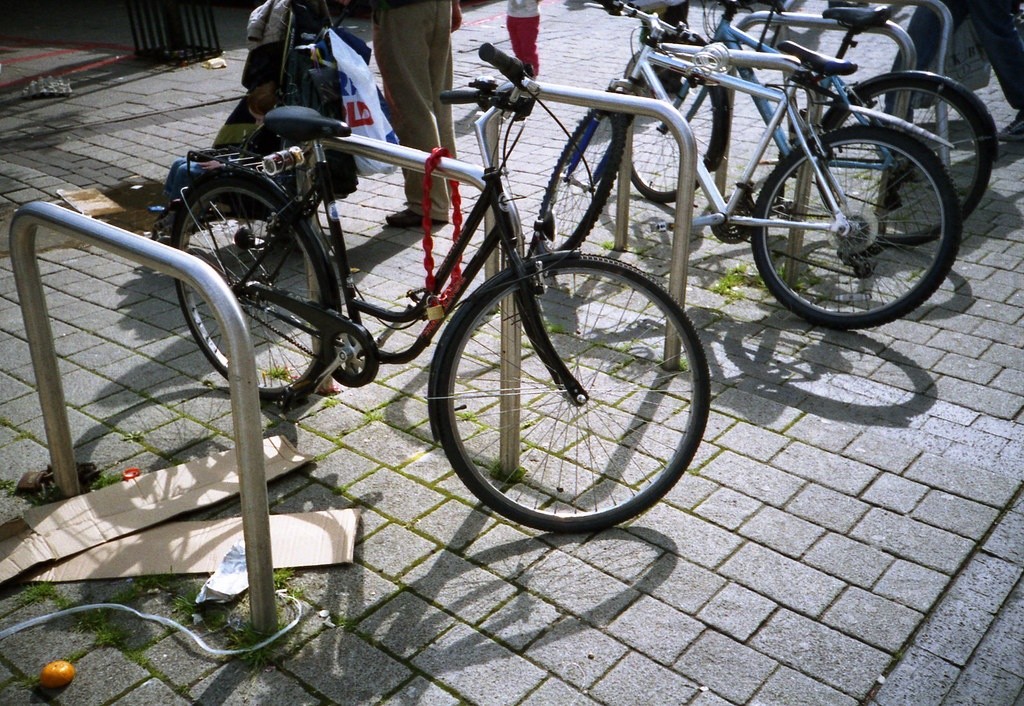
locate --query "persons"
[506,0,544,76]
[153,83,278,237]
[633,0,689,96]
[366,0,462,227]
[885,0,1024,142]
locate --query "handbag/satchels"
[910,15,991,109]
[308,50,357,197]
[324,28,400,175]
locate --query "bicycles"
[151,40,713,538]
[539,1,1002,332]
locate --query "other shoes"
[386,210,448,227]
[153,211,196,235]
[999,115,1024,141]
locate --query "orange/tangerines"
[41,661,75,688]
